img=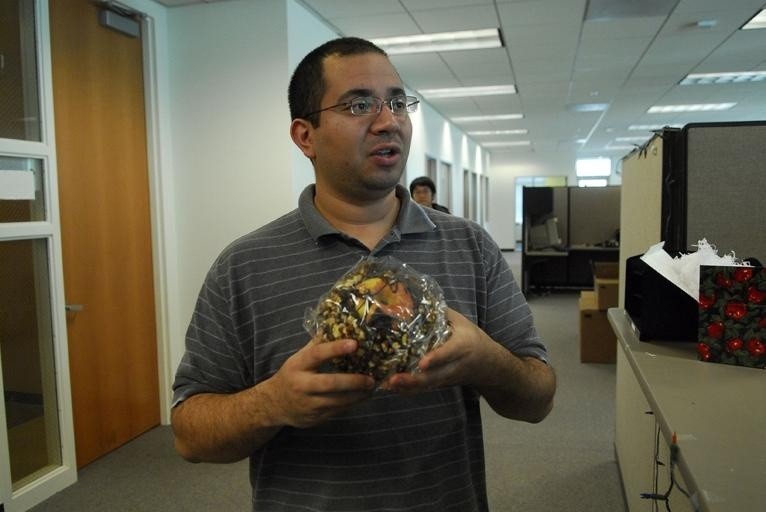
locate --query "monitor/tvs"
[529,216,562,251]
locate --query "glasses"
[302,95,420,116]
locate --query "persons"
[408,174,454,216]
[166,33,559,511]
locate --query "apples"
[348,275,415,330]
[698,268,766,360]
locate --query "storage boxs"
[579,272,619,364]
